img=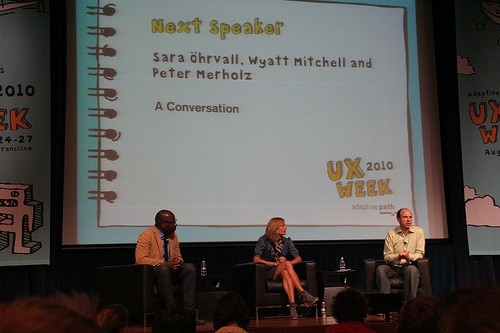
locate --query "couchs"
[95,264,200,326]
[234,261,317,319]
[363,258,432,311]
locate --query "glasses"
[162,218,177,224]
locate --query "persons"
[375,207,425,310]
[151,304,196,333]
[253,216,319,319]
[60,291,103,328]
[396,300,443,333]
[97,302,129,332]
[215,321,249,332]
[0,295,101,333]
[322,287,378,333]
[134,209,204,324]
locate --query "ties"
[164,234,169,261]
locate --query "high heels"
[289,302,299,319]
[299,290,319,309]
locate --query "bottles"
[339,257,346,270]
[200,261,207,277]
[321,302,327,318]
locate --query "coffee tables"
[317,271,355,316]
[201,276,235,321]
